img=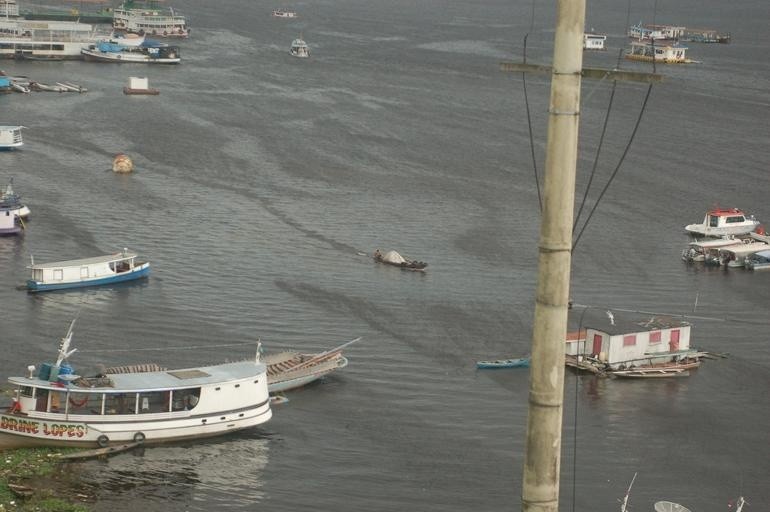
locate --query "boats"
[582,20,733,66]
[0,126,30,235]
[0,0,190,96]
[25,247,151,292]
[272,7,312,58]
[369,251,429,271]
[477,358,529,368]
[680,206,770,270]
[112,155,134,174]
